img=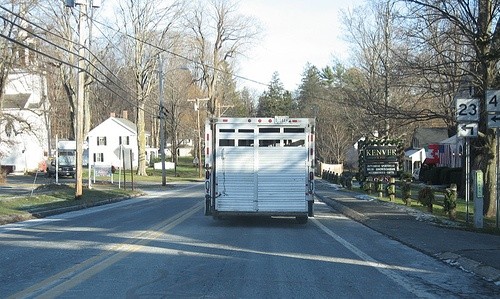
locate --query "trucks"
[47,150,76,179]
[204,115,316,223]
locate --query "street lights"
[158,52,190,186]
